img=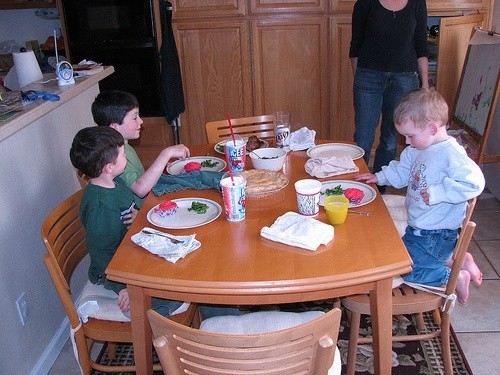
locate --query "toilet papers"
[11,49,43,88]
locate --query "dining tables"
[106,138,412,375]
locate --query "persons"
[91,90,190,209]
[349,0,429,195]
[70,126,240,320]
[355,87,485,305]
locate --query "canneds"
[273,125,290,154]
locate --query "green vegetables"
[216,144,224,152]
[201,159,219,168]
[326,185,343,195]
[188,201,208,214]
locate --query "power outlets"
[17,292,33,324]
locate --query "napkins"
[304,153,359,179]
[153,172,225,196]
[260,209,335,251]
[131,226,201,263]
[289,127,315,150]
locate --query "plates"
[165,155,227,172]
[306,142,365,161]
[146,197,223,230]
[240,169,290,198]
[318,180,377,208]
[214,137,269,155]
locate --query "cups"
[272,111,291,150]
[294,178,322,217]
[219,170,246,222]
[225,134,246,174]
[324,196,349,225]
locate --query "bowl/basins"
[249,147,287,172]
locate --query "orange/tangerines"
[44,35,65,49]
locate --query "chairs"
[147,308,342,375]
[41,188,199,375]
[205,114,276,143]
[342,197,478,375]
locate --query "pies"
[240,169,289,198]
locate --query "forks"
[142,230,184,244]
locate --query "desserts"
[343,188,365,205]
[184,162,201,173]
[158,200,178,211]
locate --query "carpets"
[92,296,474,375]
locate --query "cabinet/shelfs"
[154,0,494,148]
